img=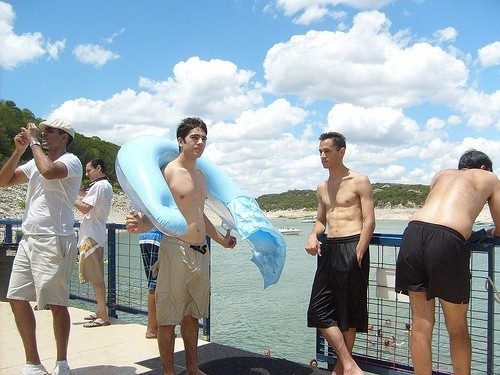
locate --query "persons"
[75,158,113,327]
[0,117,82,375]
[138,225,163,338]
[126,117,237,375]
[305,132,377,375]
[263,319,412,375]
[395,150,500,375]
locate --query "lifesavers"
[114,130,287,291]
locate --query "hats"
[39,117,76,140]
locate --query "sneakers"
[51,363,70,375]
[19,363,48,375]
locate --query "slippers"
[84,314,97,320]
[83,319,111,327]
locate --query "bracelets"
[30,141,41,147]
